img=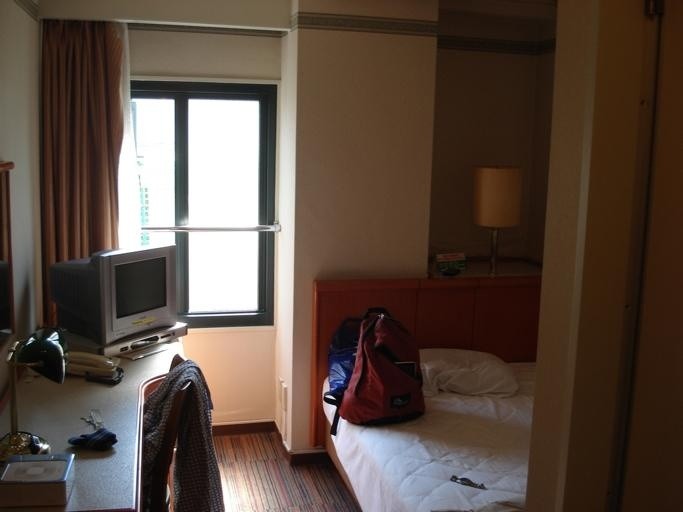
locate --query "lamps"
[0,326,72,469]
[473,164,523,275]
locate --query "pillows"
[418,347,520,399]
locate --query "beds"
[310,277,543,512]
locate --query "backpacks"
[323,316,364,437]
[337,306,426,426]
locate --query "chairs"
[141,354,196,512]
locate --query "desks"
[0,334,185,512]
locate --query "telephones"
[63,351,117,376]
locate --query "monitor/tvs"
[50,244,177,346]
